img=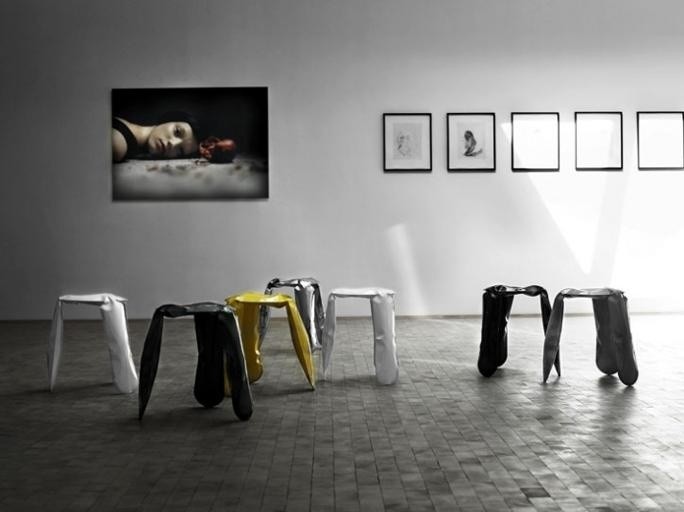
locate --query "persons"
[111,108,226,167]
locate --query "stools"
[49,292,139,396]
[254,277,324,357]
[224,290,318,393]
[321,288,398,384]
[138,301,256,421]
[476,284,639,387]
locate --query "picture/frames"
[447,113,496,170]
[637,113,684,171]
[510,114,560,170]
[574,113,623,170]
[383,113,432,172]
